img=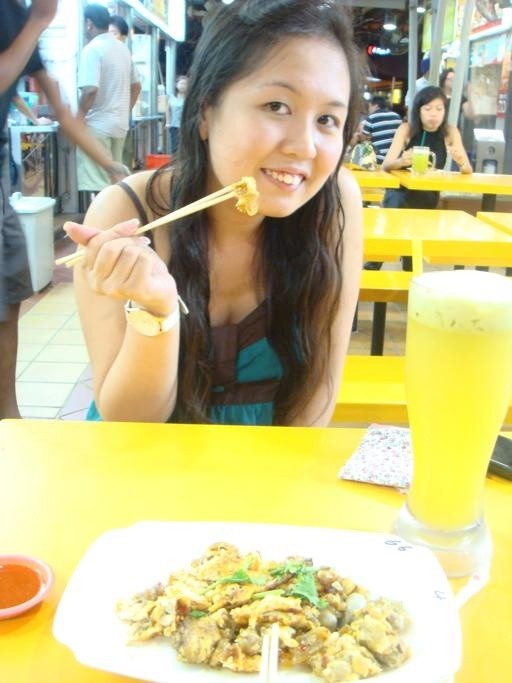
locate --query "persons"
[71,3,142,204]
[0,0,130,419]
[106,12,130,42]
[165,75,187,156]
[59,0,365,428]
[360,84,476,272]
[347,62,476,164]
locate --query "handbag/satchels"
[349,143,377,171]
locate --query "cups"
[402,269,511,530]
[412,146,436,174]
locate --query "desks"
[0,146,512,678]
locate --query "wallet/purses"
[340,423,413,488]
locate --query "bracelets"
[123,298,190,336]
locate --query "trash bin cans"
[8,192,56,294]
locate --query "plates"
[0,555,54,622]
[50,522,462,683]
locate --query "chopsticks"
[259,623,278,683]
[53,179,240,268]
